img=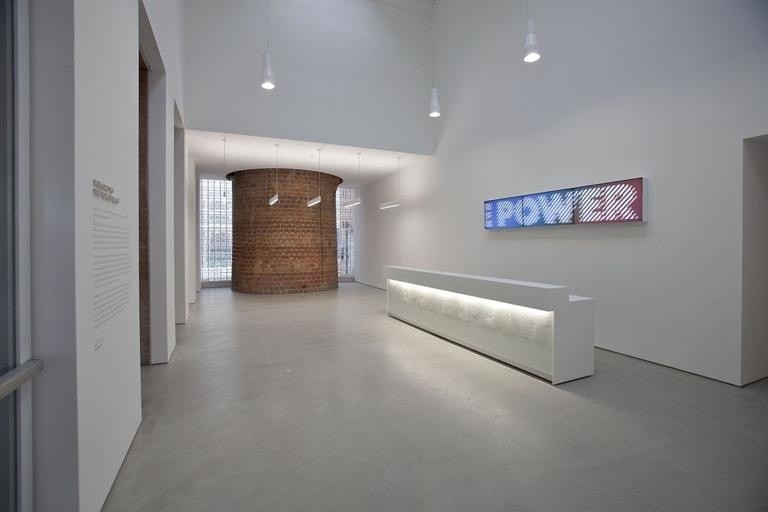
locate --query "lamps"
[305,148,325,207]
[374,156,406,209]
[258,0,276,89]
[429,0,441,117]
[519,0,542,63]
[267,144,279,205]
[337,152,362,210]
